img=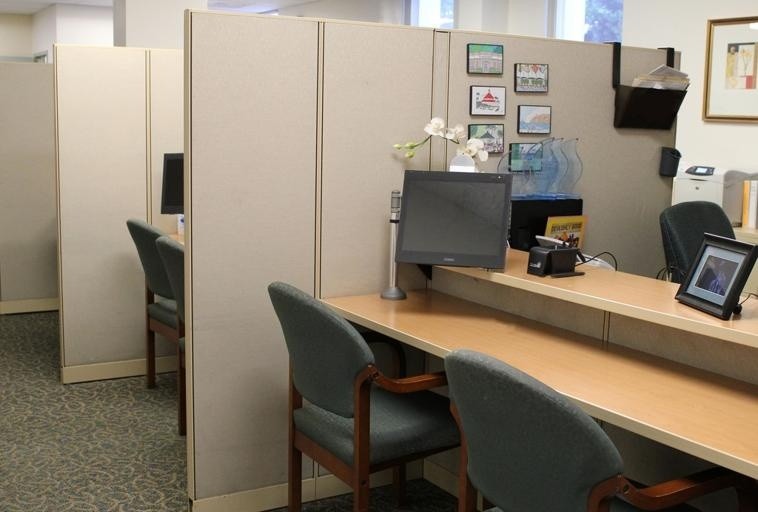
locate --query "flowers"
[393,117,489,173]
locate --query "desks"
[317,248,758,480]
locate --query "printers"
[671,164,758,227]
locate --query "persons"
[557,231,574,244]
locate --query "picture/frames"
[702,15,758,124]
[467,43,552,172]
[674,232,758,321]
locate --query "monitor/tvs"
[394,169,513,270]
[161,152,183,213]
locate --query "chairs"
[127,220,176,390]
[659,201,736,284]
[444,350,758,512]
[156,235,186,435]
[268,286,460,512]
